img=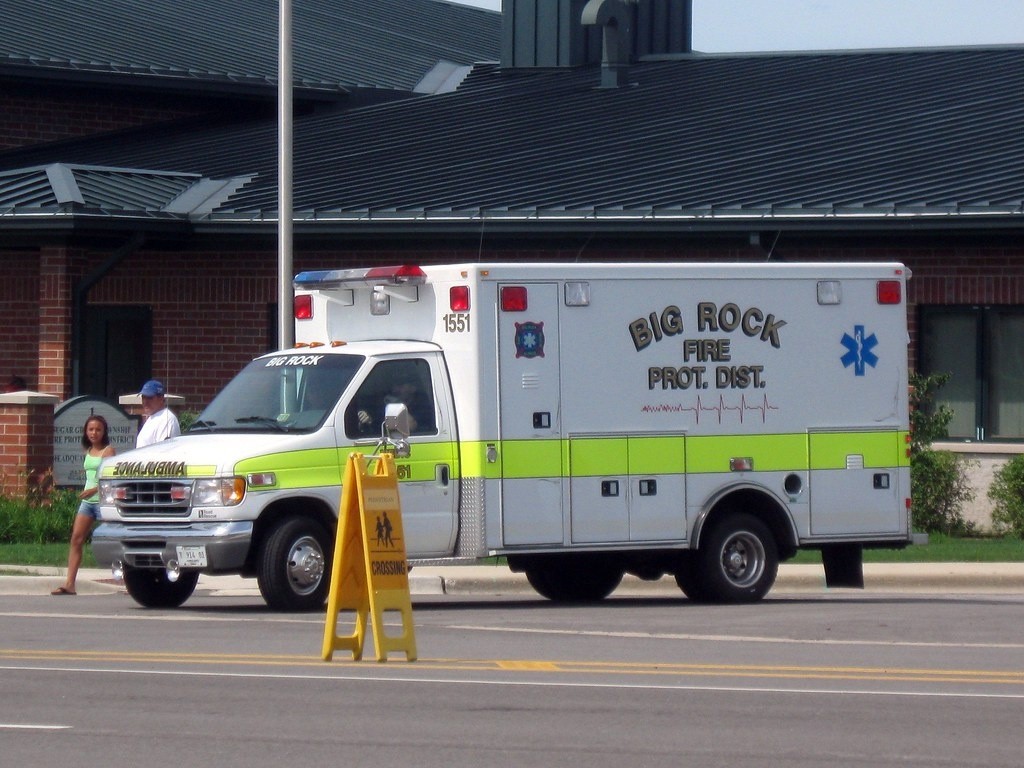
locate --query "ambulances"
[91,262,912,609]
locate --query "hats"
[388,371,419,384]
[137,380,166,398]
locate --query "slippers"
[50,585,77,596]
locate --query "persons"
[302,375,340,411]
[52,415,116,595]
[136,381,181,449]
[372,370,432,432]
